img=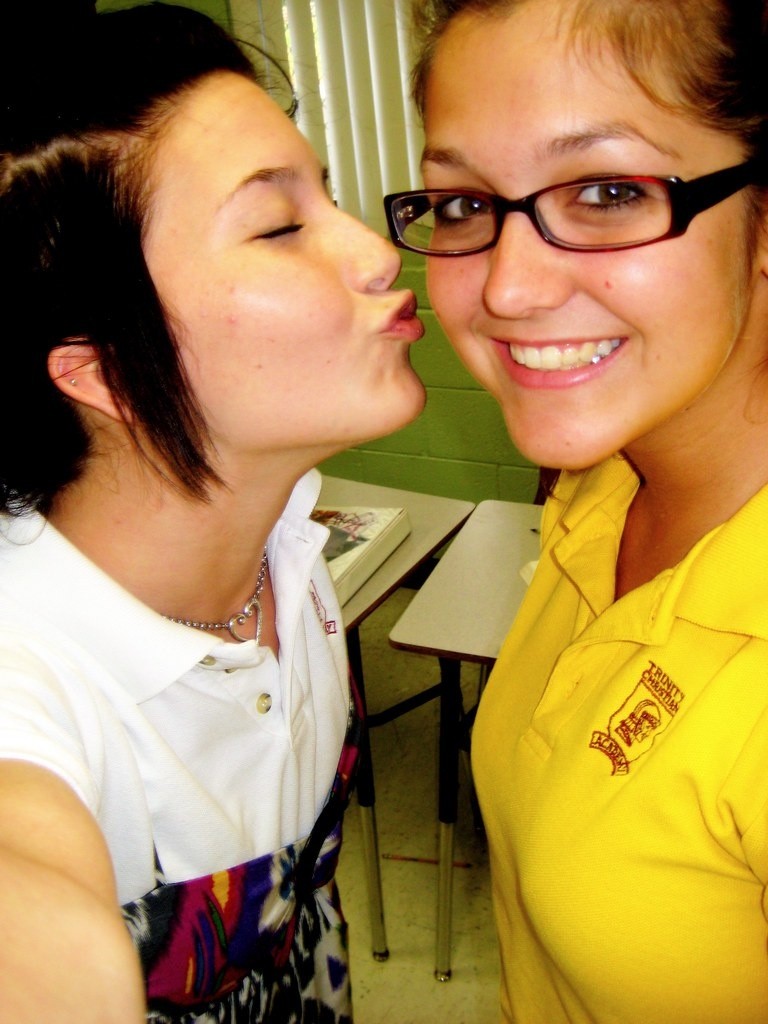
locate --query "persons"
[383,0,768,1024]
[0,0,428,1024]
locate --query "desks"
[284,473,543,983]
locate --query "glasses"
[383,157,754,254]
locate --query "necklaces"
[163,551,268,647]
